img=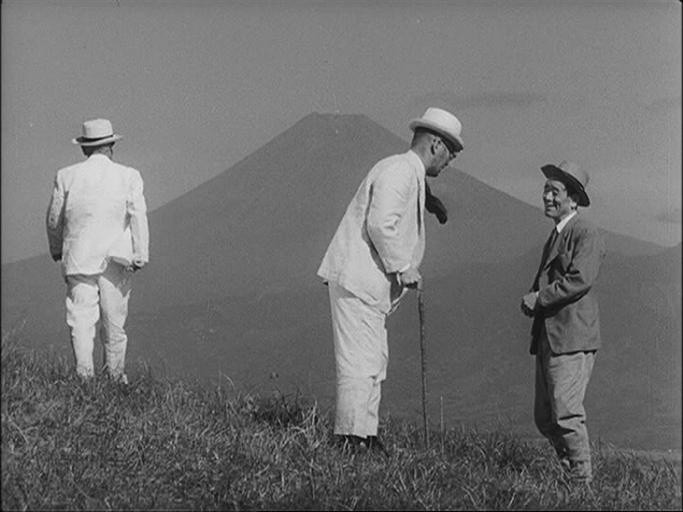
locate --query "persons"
[315,106,465,460]
[517,157,606,488]
[45,118,150,384]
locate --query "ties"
[549,230,556,251]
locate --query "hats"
[540,159,591,207]
[71,118,122,147]
[408,107,464,152]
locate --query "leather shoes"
[339,436,388,456]
